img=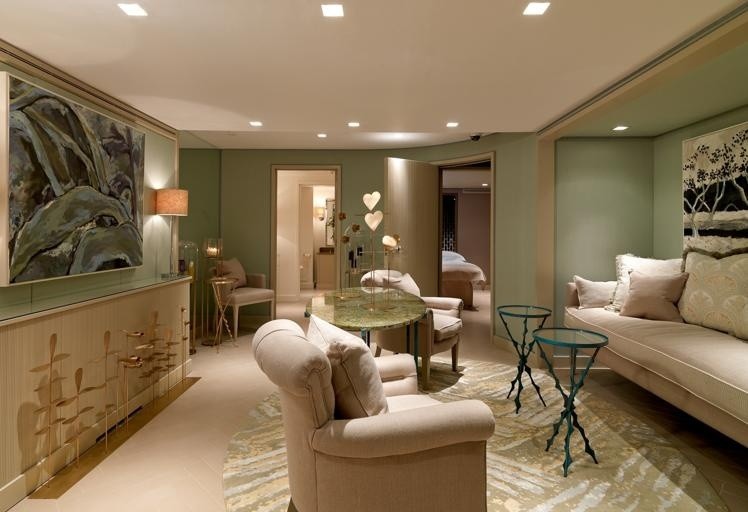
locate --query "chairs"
[359,268,465,393]
[249,315,497,512]
[208,266,275,340]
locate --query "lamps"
[154,188,189,280]
[203,237,222,259]
[315,207,325,221]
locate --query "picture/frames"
[0,71,147,290]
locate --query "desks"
[205,276,240,353]
[303,285,428,355]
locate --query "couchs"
[564,279,747,451]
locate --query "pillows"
[303,314,390,419]
[213,257,248,288]
[604,253,686,316]
[441,251,465,263]
[381,273,420,298]
[620,267,689,324]
[676,246,747,341]
[571,274,618,311]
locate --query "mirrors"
[325,199,335,246]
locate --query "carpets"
[222,357,729,512]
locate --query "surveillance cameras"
[470,133,481,142]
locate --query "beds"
[440,261,486,308]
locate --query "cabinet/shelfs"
[0,275,193,511]
[316,252,337,289]
